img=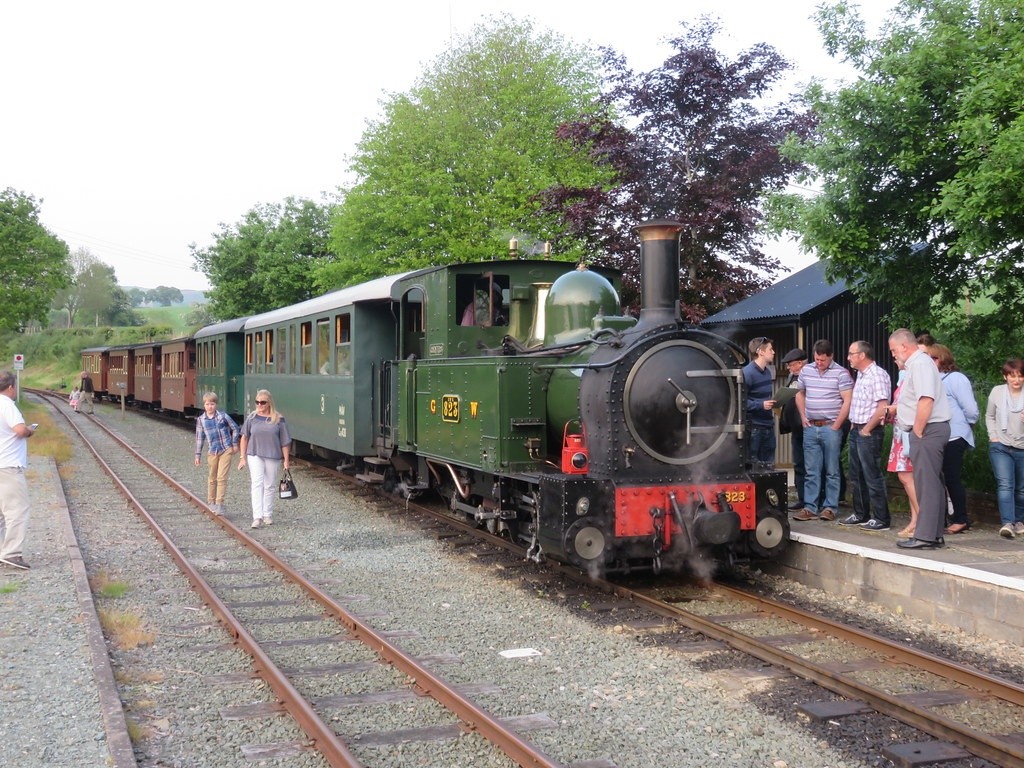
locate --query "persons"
[68,371,95,414]
[0,370,35,568]
[320,349,349,375]
[984,358,1024,538]
[742,336,777,464]
[238,389,292,528]
[460,280,503,326]
[195,392,240,515]
[782,328,979,550]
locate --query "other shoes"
[86,411,95,415]
[207,503,224,516]
[788,504,804,512]
[264,518,273,524]
[948,523,967,532]
[897,529,914,538]
[74,410,81,414]
[1000,522,1024,539]
[251,519,261,528]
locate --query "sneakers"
[793,510,818,520]
[0,557,30,570]
[859,518,890,531]
[820,509,837,520]
[839,514,870,526]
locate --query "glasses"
[756,337,768,351]
[847,351,862,357]
[255,401,269,405]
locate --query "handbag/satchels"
[279,467,297,499]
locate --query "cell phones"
[883,408,889,421]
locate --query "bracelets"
[28,430,32,438]
[239,455,245,459]
[861,429,871,437]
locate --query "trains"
[80,215,793,581]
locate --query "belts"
[809,420,829,426]
[853,423,865,428]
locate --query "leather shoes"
[934,537,944,547]
[897,539,934,550]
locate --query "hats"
[781,349,805,362]
[493,283,502,296]
